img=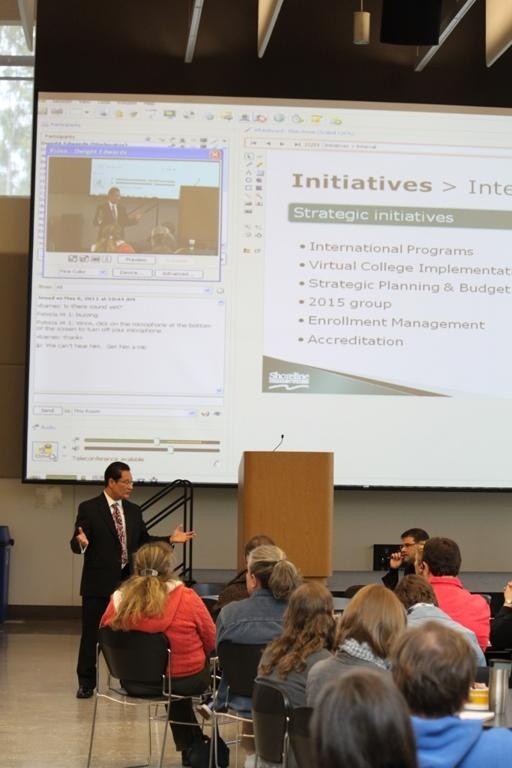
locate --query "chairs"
[461,593,512,731]
[86,627,208,768]
[249,676,292,768]
[281,705,316,767]
[207,638,273,768]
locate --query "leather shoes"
[77,686,94,697]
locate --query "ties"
[112,503,127,564]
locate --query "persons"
[92,187,180,255]
[209,529,511,767]
[69,460,198,699]
[99,540,216,765]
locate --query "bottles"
[489,667,509,716]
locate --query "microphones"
[271,432,285,452]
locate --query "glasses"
[399,543,416,547]
[116,479,135,484]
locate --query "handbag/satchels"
[183,735,229,767]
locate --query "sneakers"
[195,698,215,718]
